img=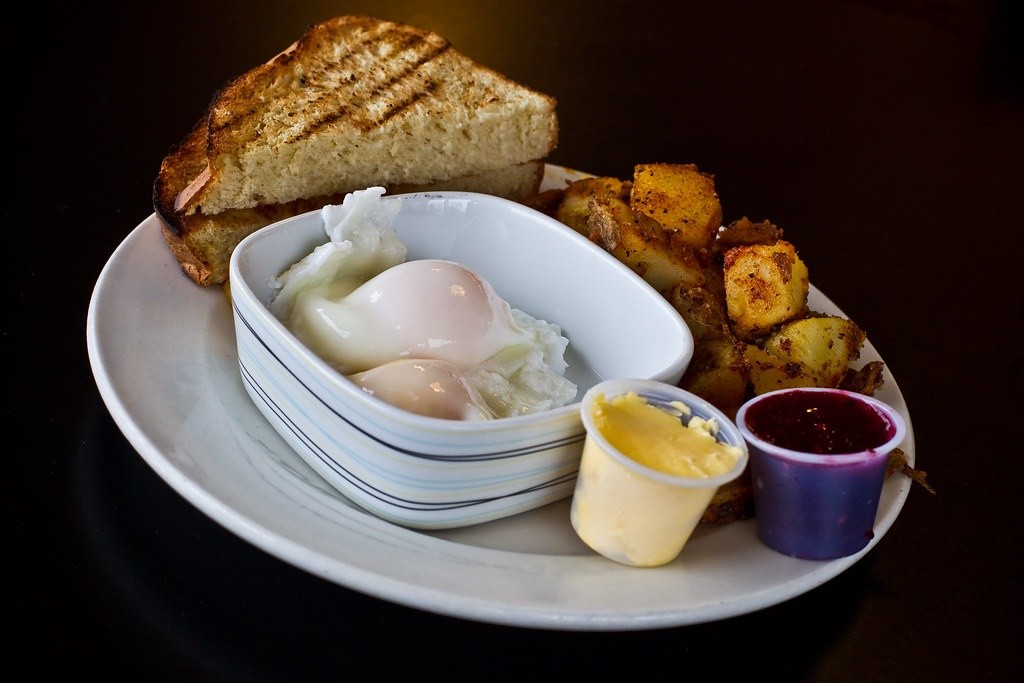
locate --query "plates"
[89,158,917,636]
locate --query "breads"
[151,13,567,280]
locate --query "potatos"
[568,163,884,429]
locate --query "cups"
[735,388,907,566]
[565,378,746,568]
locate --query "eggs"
[275,186,576,426]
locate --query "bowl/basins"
[228,190,694,530]
